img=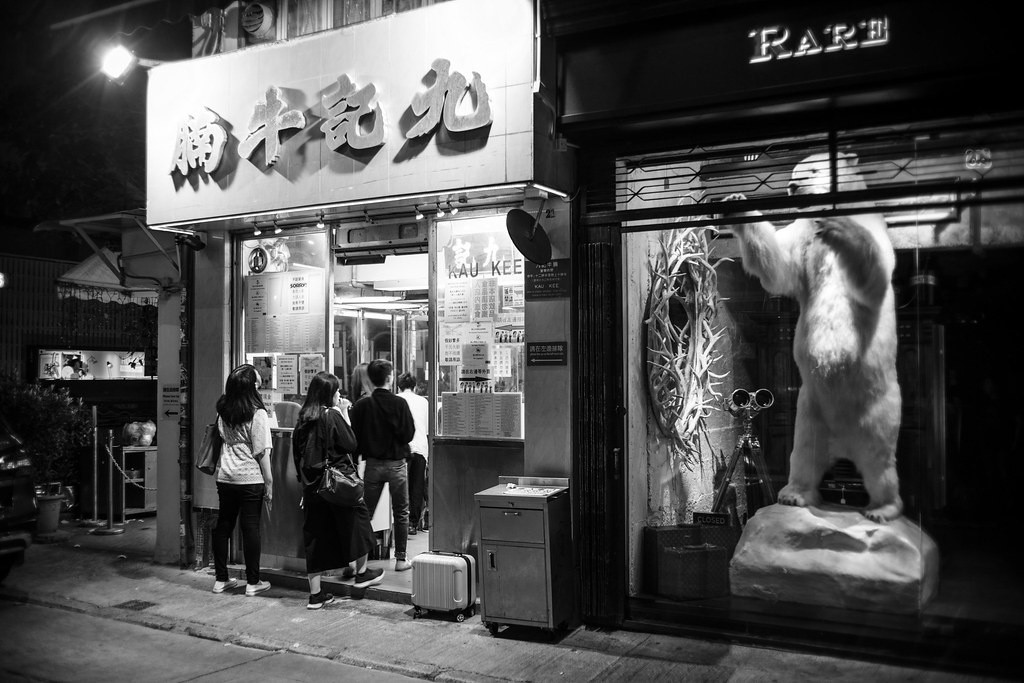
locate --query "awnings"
[32,208,179,280]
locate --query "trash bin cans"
[35,493,64,533]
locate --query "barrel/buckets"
[36,493,64,532]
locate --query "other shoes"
[340,574,356,581]
[408,524,417,535]
[395,558,411,570]
[422,525,429,533]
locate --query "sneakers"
[307,592,335,609]
[246,580,270,596]
[213,578,238,593]
[354,568,385,588]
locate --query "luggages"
[410,550,476,622]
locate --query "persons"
[350,362,375,403]
[212,364,273,597]
[291,371,386,608]
[394,372,428,535]
[342,358,412,577]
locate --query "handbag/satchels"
[196,413,223,476]
[316,466,365,504]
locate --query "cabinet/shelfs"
[101,446,157,521]
[474,475,572,641]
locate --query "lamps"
[273,220,282,234]
[316,214,324,229]
[414,205,423,221]
[445,201,458,215]
[436,202,445,218]
[253,222,261,235]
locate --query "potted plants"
[0,370,94,532]
